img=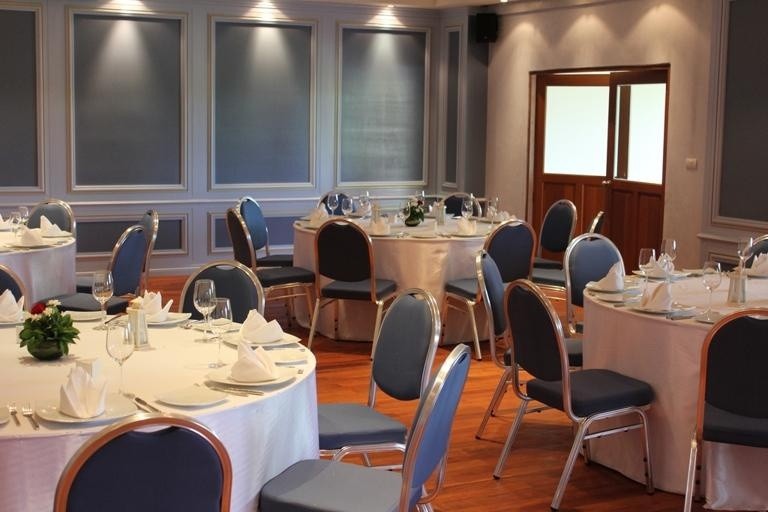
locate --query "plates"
[1,405,11,426]
[585,264,767,324]
[37,397,136,425]
[0,219,72,252]
[150,312,192,325]
[161,329,307,407]
[300,207,516,240]
[64,309,104,322]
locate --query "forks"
[22,400,45,430]
[8,401,24,427]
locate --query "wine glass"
[193,278,217,342]
[414,190,425,202]
[459,200,475,223]
[7,207,30,245]
[106,320,138,389]
[398,200,412,236]
[638,248,657,297]
[660,239,678,261]
[91,270,113,331]
[205,297,233,369]
[323,190,372,220]
[701,261,723,322]
[736,236,754,268]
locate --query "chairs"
[474,249,582,440]
[739,230,768,270]
[490,278,656,511]
[315,289,443,512]
[51,410,232,511]
[684,308,768,511]
[259,342,476,512]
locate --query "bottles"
[122,303,148,346]
